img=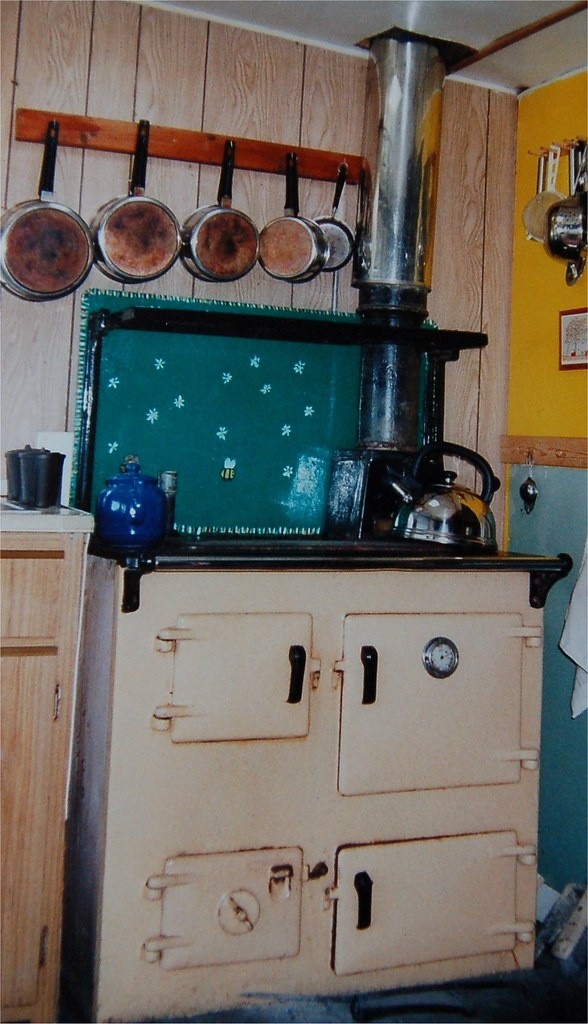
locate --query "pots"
[0,120,96,301]
[258,153,331,284]
[180,141,261,283]
[314,165,354,273]
[89,118,183,284]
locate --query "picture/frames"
[557,307,587,370]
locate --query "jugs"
[94,464,168,552]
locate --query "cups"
[5,445,49,501]
[18,449,65,509]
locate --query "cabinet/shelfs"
[82,540,575,1022]
[0,490,95,1022]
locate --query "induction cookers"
[85,538,572,578]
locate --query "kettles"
[388,439,497,555]
